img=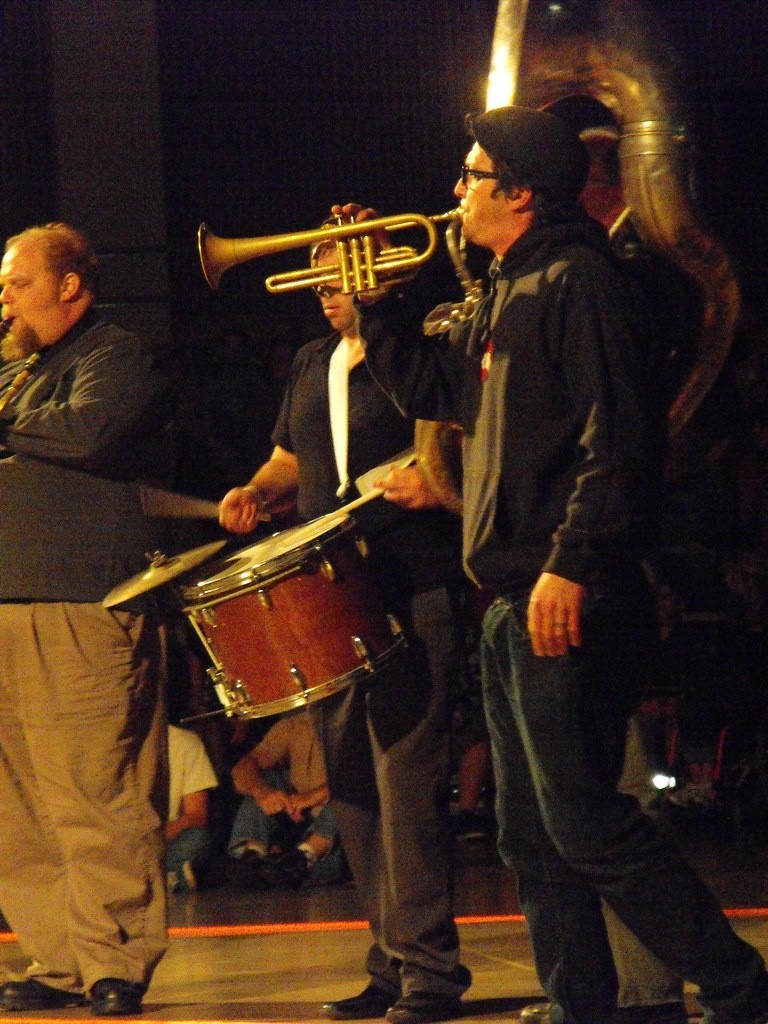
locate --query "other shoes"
[89,978,142,1015]
[384,998,464,1024]
[320,986,396,1018]
[516,997,554,1023]
[238,849,300,888]
[170,874,185,896]
[0,978,88,1010]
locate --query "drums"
[178,501,411,721]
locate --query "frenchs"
[462,0,741,446]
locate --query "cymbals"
[101,541,229,608]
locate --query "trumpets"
[196,203,466,299]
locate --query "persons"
[0,224,168,1017]
[163,108,768,1024]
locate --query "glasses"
[459,164,501,186]
